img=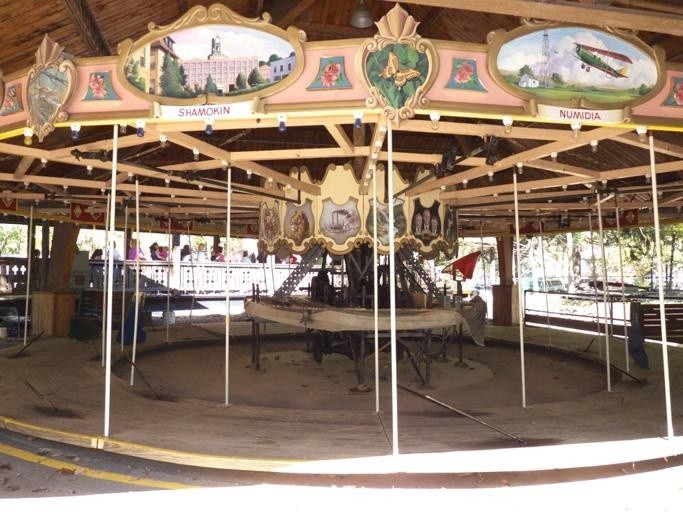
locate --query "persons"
[414,210,438,240]
[90,238,303,265]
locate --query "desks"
[30,290,82,338]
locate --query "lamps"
[550,153,559,163]
[503,118,514,134]
[349,0,373,28]
[590,140,600,153]
[570,122,583,139]
[363,125,386,187]
[636,126,650,141]
[493,174,662,227]
[429,116,441,130]
[220,160,292,195]
[4,118,213,225]
[277,111,288,132]
[440,163,524,192]
[353,114,362,129]
